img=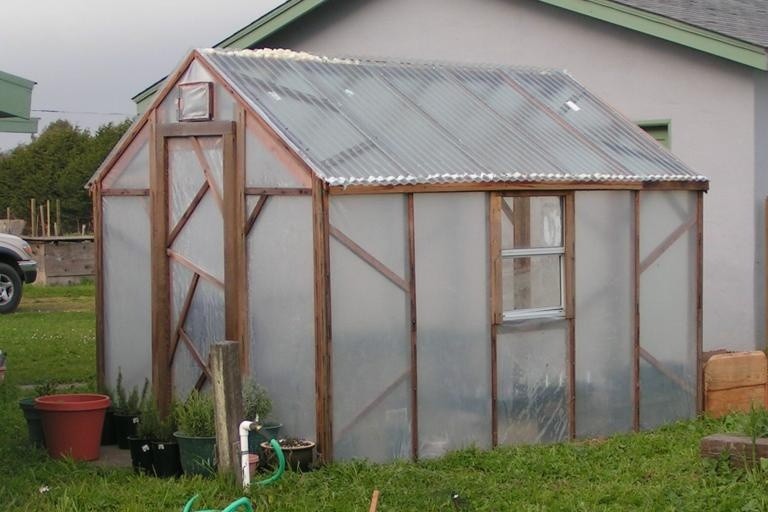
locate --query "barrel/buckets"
[33,393,113,460]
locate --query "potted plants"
[101,362,282,480]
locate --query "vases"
[248,438,315,476]
[19,392,112,460]
